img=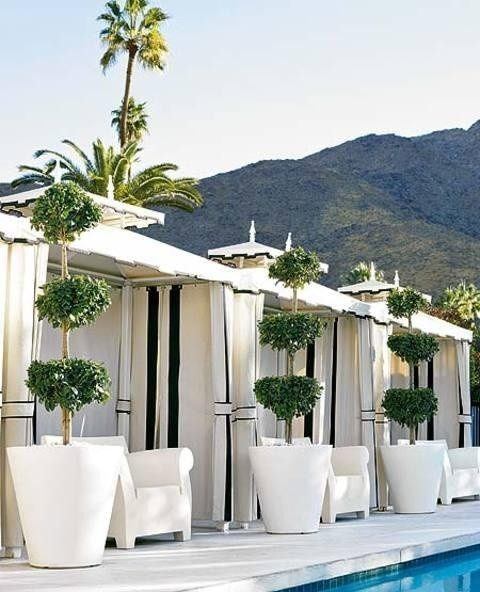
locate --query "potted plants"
[379,286,446,514]
[248,245,333,534]
[6,180,124,569]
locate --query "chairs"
[398,439,480,505]
[42,435,193,549]
[259,437,370,524]
